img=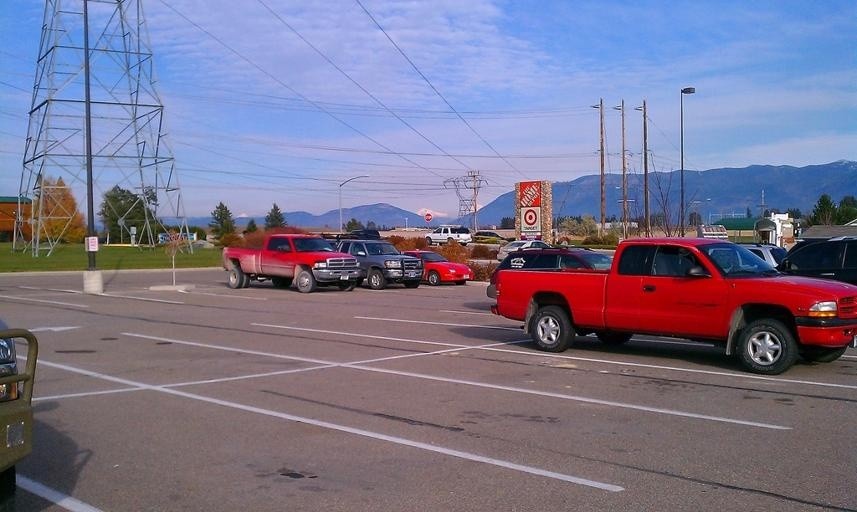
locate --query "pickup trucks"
[222,233,362,294]
[475,237,857,376]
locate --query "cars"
[425,224,473,246]
[399,249,475,287]
[487,240,612,299]
[472,231,506,244]
[736,235,857,286]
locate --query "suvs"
[319,232,425,290]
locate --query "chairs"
[656,255,687,277]
[714,250,740,273]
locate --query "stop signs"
[425,213,432,222]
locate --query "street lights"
[680,87,697,238]
[339,174,370,233]
